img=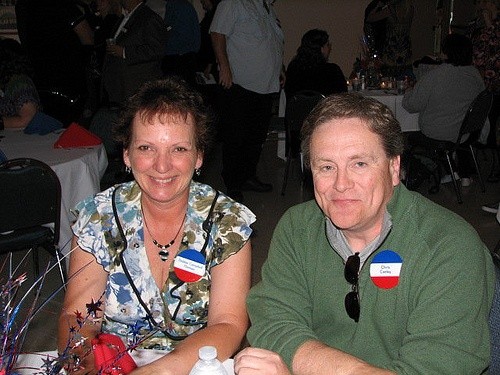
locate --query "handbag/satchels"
[92,332,138,375]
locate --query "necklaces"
[140,191,187,262]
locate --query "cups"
[106,38,117,48]
[352,70,415,95]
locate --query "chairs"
[88,106,122,192]
[0,157,68,298]
[40,91,72,129]
[400,77,500,205]
[280,89,326,196]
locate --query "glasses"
[343,251,360,323]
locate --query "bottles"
[189,346,228,375]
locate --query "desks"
[0,127,110,278]
[0,347,237,375]
[347,89,421,186]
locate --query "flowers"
[471,19,500,81]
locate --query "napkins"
[55,122,102,148]
[23,110,64,136]
[91,332,139,375]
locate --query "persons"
[284,28,349,158]
[0,0,286,207]
[233,91,496,375]
[364,0,500,265]
[56,76,257,375]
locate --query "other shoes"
[243,178,272,192]
[228,190,244,202]
